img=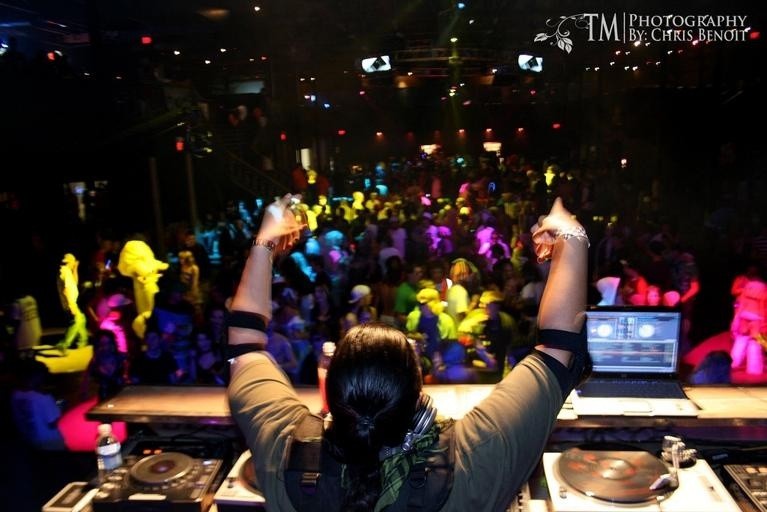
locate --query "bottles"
[316,341,337,413]
[96,424,123,483]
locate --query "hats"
[61,254,75,264]
[348,284,371,304]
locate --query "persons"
[0,151,767,512]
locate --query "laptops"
[569,307,698,419]
